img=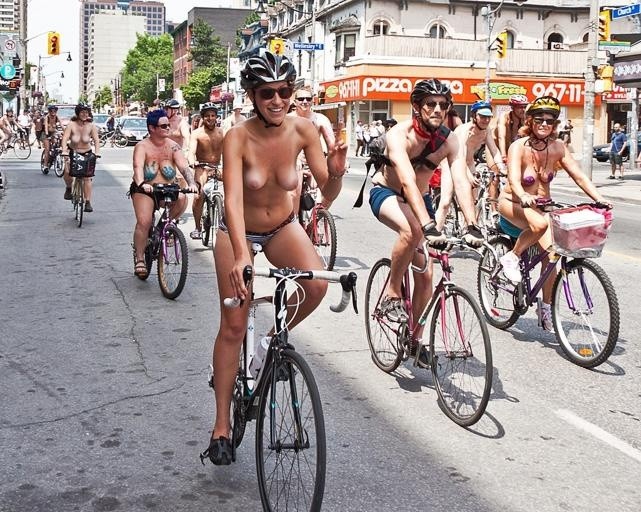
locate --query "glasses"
[425,100,450,110]
[156,123,170,129]
[255,87,294,99]
[295,97,312,101]
[534,118,555,125]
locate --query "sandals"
[134,259,148,274]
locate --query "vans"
[48,104,78,134]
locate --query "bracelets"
[327,171,347,181]
[139,180,146,188]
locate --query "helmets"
[200,101,219,112]
[6,108,13,112]
[471,101,493,112]
[165,100,181,108]
[525,95,561,117]
[240,51,297,90]
[410,79,452,106]
[509,94,528,105]
[74,103,91,116]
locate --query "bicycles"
[1,129,32,160]
[91,123,129,147]
[41,125,102,228]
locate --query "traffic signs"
[613,4,640,19]
[293,42,323,50]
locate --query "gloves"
[461,224,485,249]
[421,220,449,250]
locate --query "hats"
[233,104,243,111]
[477,107,494,117]
[48,105,58,111]
[382,118,397,128]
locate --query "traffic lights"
[599,66,615,92]
[50,34,60,55]
[475,90,485,102]
[497,33,508,59]
[600,11,610,41]
[271,39,282,55]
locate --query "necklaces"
[531,138,550,176]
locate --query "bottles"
[248,335,274,380]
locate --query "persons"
[223,102,249,140]
[434,99,507,229]
[369,77,487,370]
[286,86,335,221]
[495,96,611,335]
[609,122,627,180]
[188,102,225,240]
[355,118,398,157]
[209,48,347,464]
[190,114,204,132]
[487,93,529,217]
[160,96,191,156]
[130,109,201,279]
[216,116,222,127]
[441,100,462,131]
[1,96,118,213]
[562,118,573,147]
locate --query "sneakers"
[7,145,14,149]
[407,340,432,370]
[84,200,92,212]
[190,228,203,239]
[209,423,234,466]
[535,299,557,334]
[607,174,624,181]
[499,249,524,283]
[380,294,410,323]
[43,166,48,169]
[64,187,73,200]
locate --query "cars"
[93,114,119,133]
[120,116,151,145]
[594,131,641,162]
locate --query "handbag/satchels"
[300,193,316,211]
[68,150,96,178]
[621,146,630,162]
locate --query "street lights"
[38,51,73,92]
[111,79,117,104]
